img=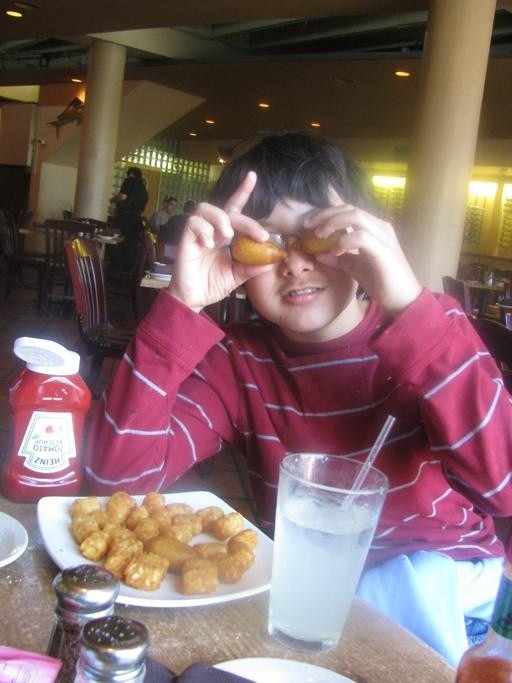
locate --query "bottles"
[45,565,121,682]
[0,336,92,501]
[71,615,147,683]
[454,557,512,683]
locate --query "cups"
[267,452,389,654]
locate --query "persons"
[84,133,511,666]
[150,196,178,233]
[109,167,149,270]
[170,201,196,232]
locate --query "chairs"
[34,208,106,300]
[442,262,511,397]
[67,238,134,388]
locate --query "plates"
[145,270,172,282]
[0,510,29,570]
[210,657,357,683]
[36,490,274,608]
